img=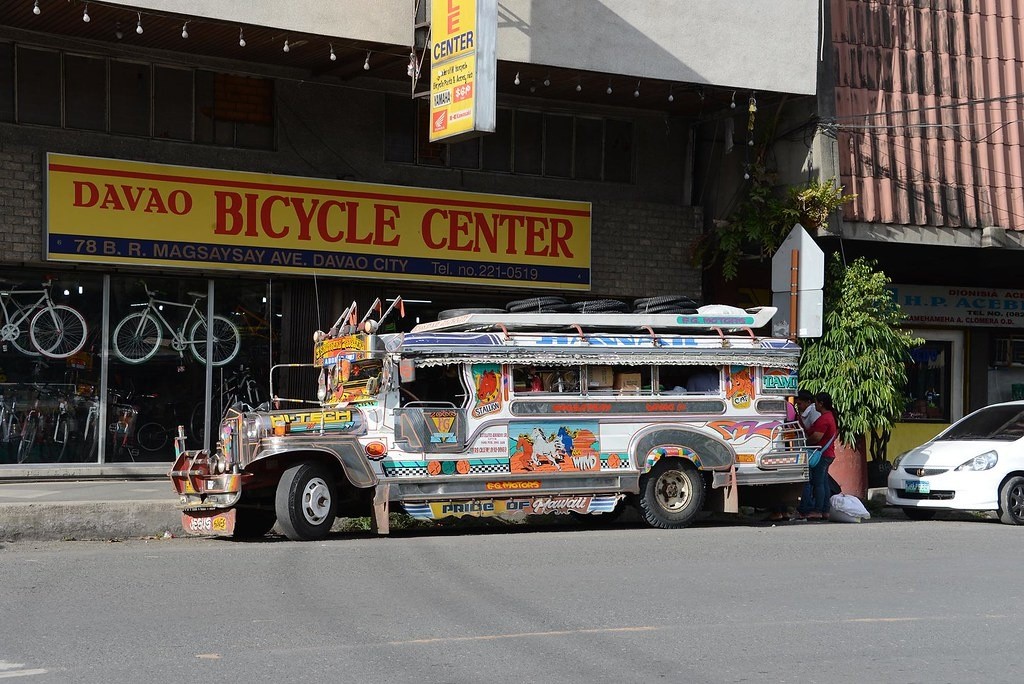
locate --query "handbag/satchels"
[807,449,822,468]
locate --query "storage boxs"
[587,365,641,396]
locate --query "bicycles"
[0,366,270,464]
[0,275,90,361]
[110,281,241,368]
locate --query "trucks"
[165,295,813,547]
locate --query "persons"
[759,390,838,522]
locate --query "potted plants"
[794,251,925,489]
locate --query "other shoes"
[758,511,830,524]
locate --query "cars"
[882,398,1024,528]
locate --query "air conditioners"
[994,338,1024,368]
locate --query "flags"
[342,297,405,326]
